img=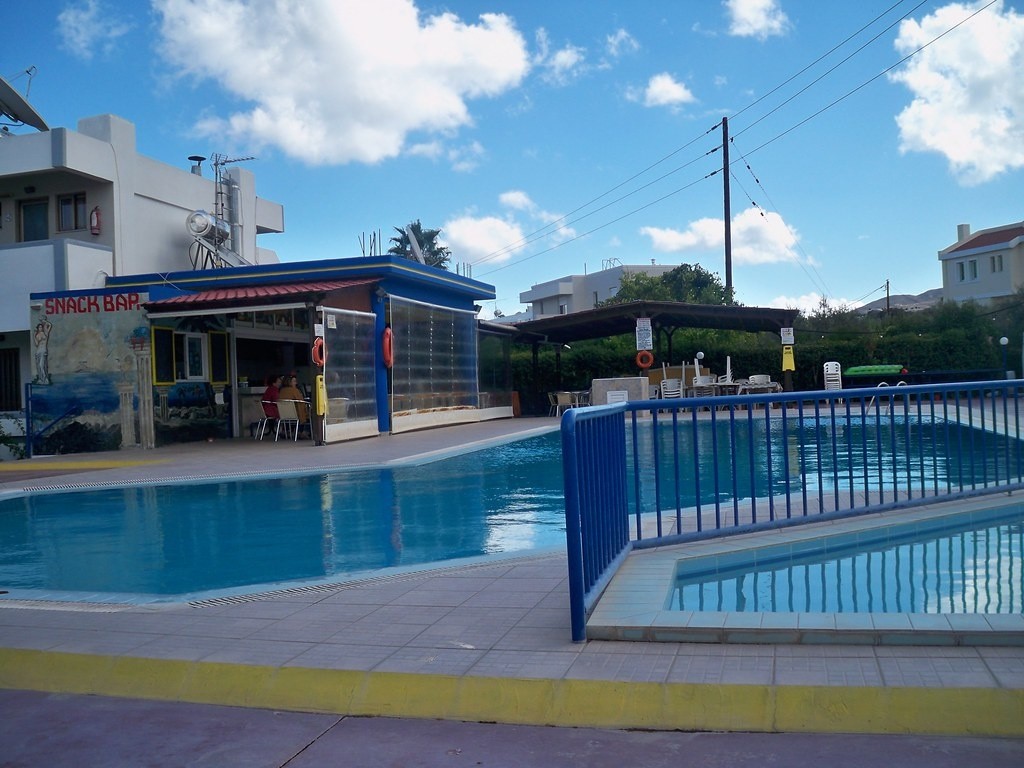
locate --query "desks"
[715,381,778,412]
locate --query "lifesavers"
[310,337,330,368]
[635,349,654,370]
[381,324,397,372]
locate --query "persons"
[261,376,291,440]
[278,375,309,440]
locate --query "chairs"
[649,385,659,414]
[255,400,314,442]
[606,391,629,404]
[660,356,772,414]
[548,388,591,417]
[823,361,843,405]
